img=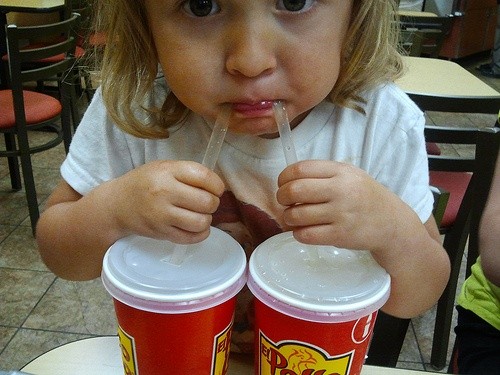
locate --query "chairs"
[395,12,463,62]
[406,92,500,371]
[393,30,441,155]
[0,0,109,238]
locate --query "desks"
[394,54,500,96]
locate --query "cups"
[101,225,248,375]
[246,229,392,374]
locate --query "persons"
[35,0,451,352]
[475,0,500,78]
[447,150,500,375]
